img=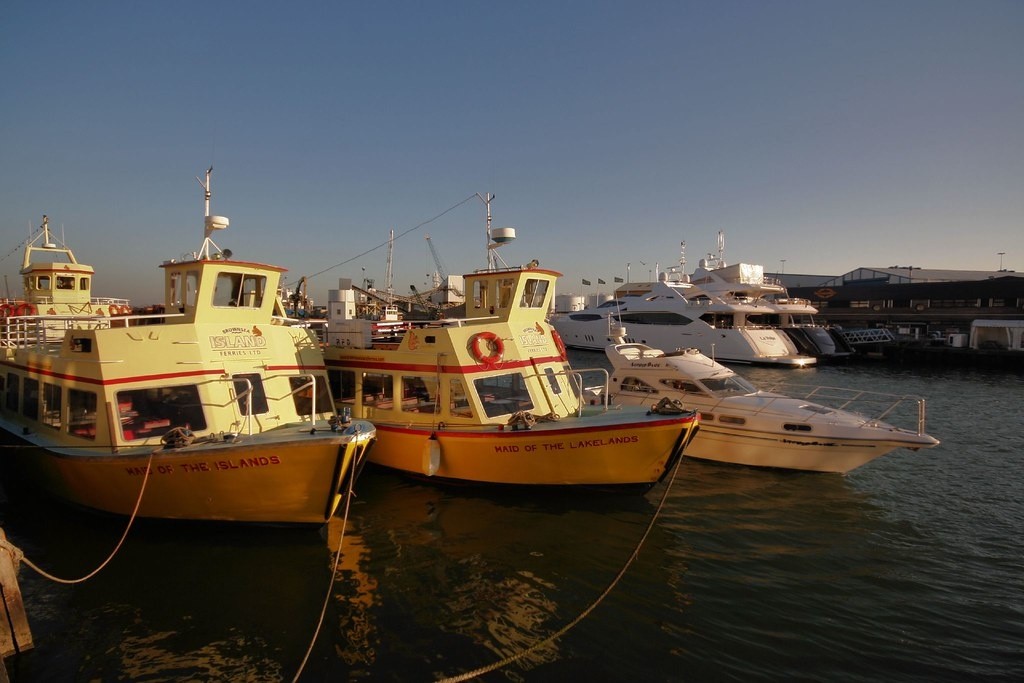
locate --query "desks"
[337,389,532,420]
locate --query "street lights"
[997,252,1005,269]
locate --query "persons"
[631,379,640,392]
[60,282,70,289]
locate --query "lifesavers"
[871,304,883,313]
[471,331,504,363]
[120,305,131,315]
[109,304,121,316]
[914,303,926,313]
[551,330,566,357]
[0,304,15,321]
[16,304,36,321]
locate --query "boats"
[1,163,857,524]
[579,289,941,474]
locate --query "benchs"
[58,400,204,443]
